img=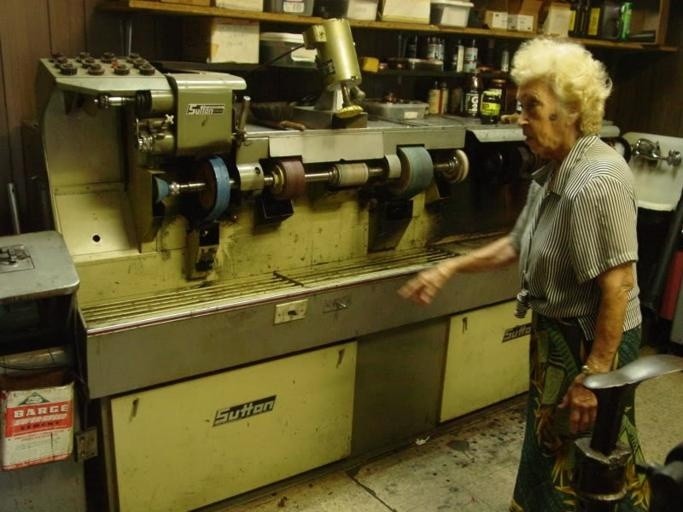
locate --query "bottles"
[568,1,633,43]
[385,33,521,124]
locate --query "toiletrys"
[618,2,634,40]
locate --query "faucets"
[633,133,662,167]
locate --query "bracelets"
[579,365,604,378]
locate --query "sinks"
[620,129,683,212]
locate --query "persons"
[395,34,653,511]
